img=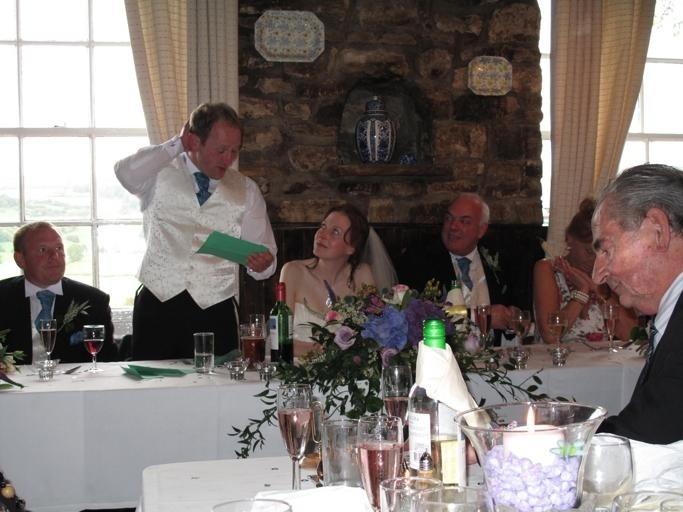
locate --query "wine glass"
[276,385,312,490]
[83,325,105,373]
[603,304,619,352]
[360,415,404,512]
[476,303,491,353]
[548,311,568,344]
[39,319,57,358]
[511,311,530,346]
[583,434,633,512]
[250,314,267,337]
[382,366,412,415]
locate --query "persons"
[1,222,119,362]
[115,103,278,360]
[280,204,399,357]
[591,163,683,444]
[534,196,640,344]
[400,193,523,328]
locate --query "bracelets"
[571,291,589,303]
[602,287,611,301]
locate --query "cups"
[224,361,250,380]
[321,420,358,486]
[379,477,490,512]
[194,333,214,373]
[253,363,276,380]
[547,345,571,366]
[34,359,60,380]
[453,403,608,511]
[240,324,249,336]
[507,347,531,369]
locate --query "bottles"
[268,281,294,366]
[446,278,464,329]
[407,319,447,475]
[418,450,436,489]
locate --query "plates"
[467,55,513,95]
[253,10,324,61]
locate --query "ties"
[34,290,54,334]
[456,258,473,292]
[194,172,212,207]
[630,321,657,402]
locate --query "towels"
[253,487,374,512]
[415,340,493,427]
[563,433,680,485]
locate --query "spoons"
[26,365,82,375]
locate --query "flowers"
[226,278,576,458]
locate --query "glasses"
[444,213,488,227]
[575,241,595,256]
